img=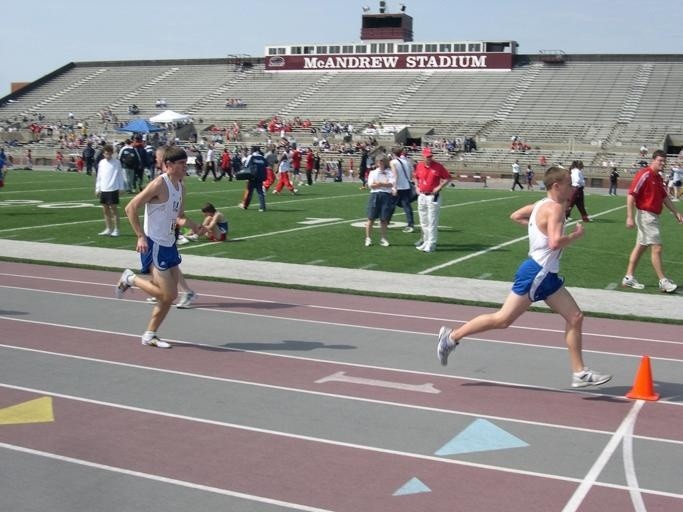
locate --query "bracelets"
[568,233,575,240]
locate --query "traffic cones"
[626,355,661,401]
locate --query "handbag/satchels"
[408,183,418,202]
[233,163,257,182]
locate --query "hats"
[423,148,433,158]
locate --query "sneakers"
[98,228,112,235]
[115,269,134,299]
[176,289,198,309]
[437,326,459,367]
[622,275,646,290]
[184,235,199,241]
[658,278,679,294]
[414,239,436,252]
[141,335,171,348]
[110,230,121,237]
[572,366,613,388]
[401,227,414,232]
[379,238,391,246]
[365,238,372,247]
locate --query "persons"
[147,146,196,309]
[183,202,230,242]
[619,150,683,293]
[608,167,619,195]
[658,161,683,202]
[115,146,188,349]
[510,159,524,192]
[364,154,393,248]
[95,146,124,238]
[1,97,476,214]
[525,165,535,191]
[510,136,531,152]
[639,145,648,156]
[389,147,414,234]
[437,166,613,389]
[566,160,593,223]
[412,148,451,253]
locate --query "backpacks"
[125,152,138,169]
